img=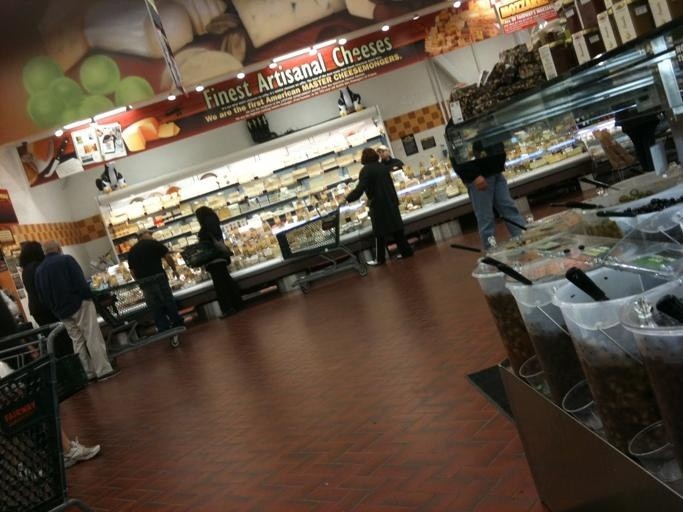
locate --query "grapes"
[22,53,155,131]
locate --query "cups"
[560,377,607,442]
[517,352,549,401]
[628,419,682,494]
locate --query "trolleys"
[0,322,100,510]
[91,275,189,368]
[275,201,372,294]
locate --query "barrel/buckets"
[472,171,683,471]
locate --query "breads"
[38,1,389,92]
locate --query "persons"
[612,100,661,173]
[127,228,184,333]
[18,240,60,338]
[0,292,102,481]
[338,147,414,266]
[34,239,121,383]
[445,88,525,250]
[195,205,245,320]
[376,145,404,172]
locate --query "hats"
[375,144,390,153]
[136,226,154,237]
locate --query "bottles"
[401,143,447,178]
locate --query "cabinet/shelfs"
[93,102,398,265]
[89,113,601,350]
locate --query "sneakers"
[396,253,414,261]
[218,307,237,320]
[365,257,384,266]
[61,440,101,470]
[87,367,122,385]
